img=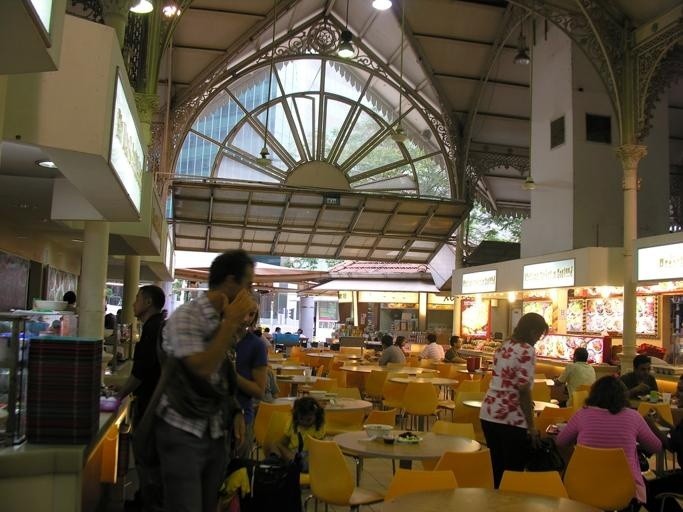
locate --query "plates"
[397,436,423,442]
[639,397,649,400]
[545,424,559,434]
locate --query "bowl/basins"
[35,300,69,311]
[362,423,394,440]
[326,392,337,400]
[309,390,327,399]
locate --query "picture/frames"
[400,322,408,331]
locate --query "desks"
[276,375,316,397]
[333,431,481,469]
[386,377,460,430]
[339,366,440,388]
[274,397,373,433]
[272,365,305,375]
[305,353,361,372]
[463,401,560,411]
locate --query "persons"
[551,346,597,407]
[418,333,446,361]
[117,286,168,509]
[297,329,307,338]
[480,313,548,490]
[378,334,407,366]
[272,327,282,336]
[557,374,661,512]
[160,308,169,319]
[226,293,267,459]
[444,336,471,363]
[645,372,683,473]
[396,336,411,357]
[262,328,272,340]
[620,354,660,401]
[271,397,328,472]
[60,291,77,311]
[142,250,256,512]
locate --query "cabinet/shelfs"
[460,347,618,401]
[14,312,79,441]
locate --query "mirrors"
[460,298,491,341]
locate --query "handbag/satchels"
[526,436,565,471]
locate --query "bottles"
[650,391,657,403]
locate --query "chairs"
[563,444,637,510]
[432,421,474,440]
[386,470,460,500]
[419,359,434,368]
[361,408,398,476]
[473,371,492,392]
[250,401,293,460]
[575,384,592,393]
[362,370,388,410]
[535,374,546,379]
[637,402,674,472]
[401,382,442,430]
[313,379,338,393]
[298,365,323,397]
[532,382,559,404]
[299,338,309,347]
[536,407,573,438]
[434,448,494,489]
[382,372,408,412]
[440,365,458,379]
[499,471,568,498]
[329,388,361,400]
[454,392,486,446]
[436,379,489,422]
[572,391,589,411]
[303,432,384,511]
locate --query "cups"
[383,427,395,445]
[304,366,312,382]
[662,392,672,405]
[650,390,657,403]
[296,388,303,399]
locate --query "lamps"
[257,0,276,167]
[338,0,354,58]
[391,0,406,143]
[522,15,537,191]
[514,5,531,65]
